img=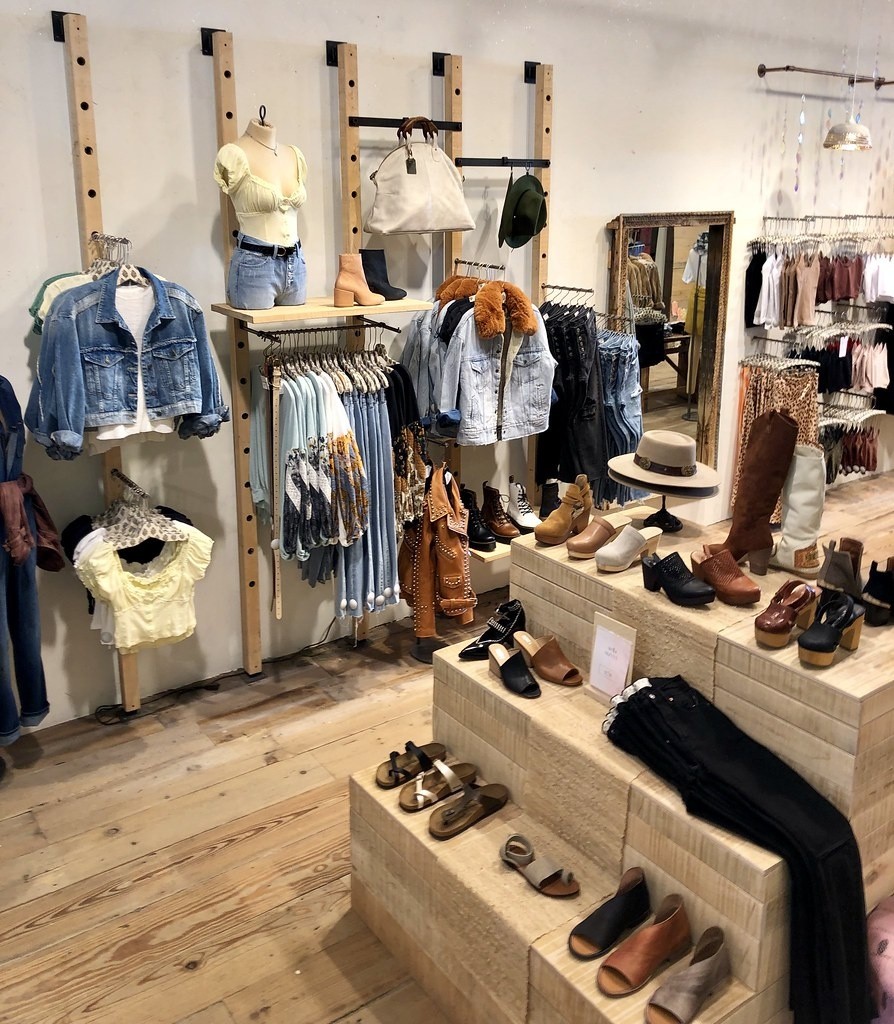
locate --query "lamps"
[822,0,877,154]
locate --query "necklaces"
[245,131,278,156]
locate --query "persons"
[219,118,310,308]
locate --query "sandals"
[498,834,581,898]
[569,866,732,1024]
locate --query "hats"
[499,174,546,247]
[606,430,721,500]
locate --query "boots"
[358,248,407,299]
[700,408,827,580]
[460,475,561,552]
[334,254,385,307]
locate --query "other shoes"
[817,537,894,625]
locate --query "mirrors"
[606,208,738,498]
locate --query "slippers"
[566,515,633,560]
[375,741,508,842]
[642,552,715,606]
[691,549,760,605]
[488,632,583,698]
[595,524,662,572]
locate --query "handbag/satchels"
[362,116,475,233]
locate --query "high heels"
[459,599,525,660]
[754,580,822,648]
[797,592,866,667]
[534,475,591,544]
